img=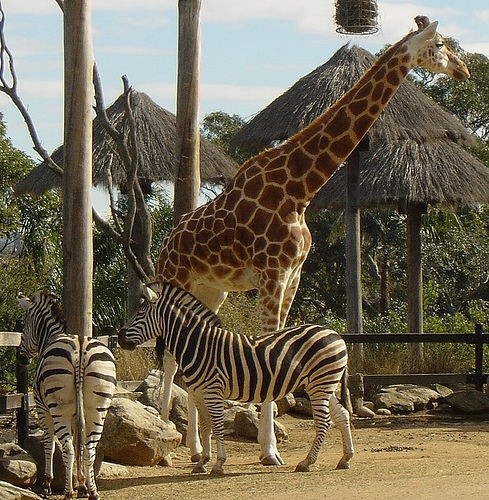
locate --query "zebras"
[117,281,354,476]
[16,288,117,500]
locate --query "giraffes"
[156,16,470,463]
